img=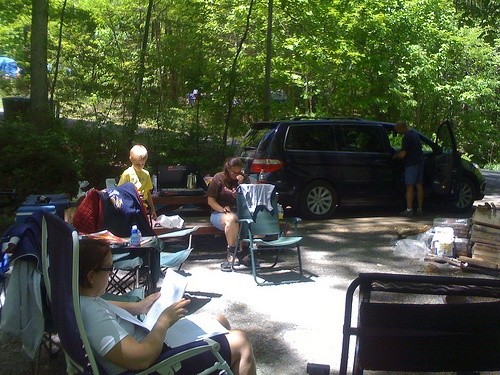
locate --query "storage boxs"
[17,194,70,222]
[432,206,500,269]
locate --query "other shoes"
[226,253,239,265]
[243,252,258,263]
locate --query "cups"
[306,363,330,375]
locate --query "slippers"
[221,261,232,272]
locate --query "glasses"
[95,265,115,274]
[230,169,243,175]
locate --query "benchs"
[82,193,288,235]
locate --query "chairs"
[307,273,500,375]
[33,183,302,375]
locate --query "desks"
[111,237,161,293]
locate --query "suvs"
[232,115,488,221]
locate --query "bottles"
[187,173,197,189]
[129,225,142,246]
[258,169,266,184]
[36,195,50,203]
[277,205,284,220]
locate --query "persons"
[78,239,257,375]
[117,144,157,224]
[394,120,423,217]
[207,157,251,266]
